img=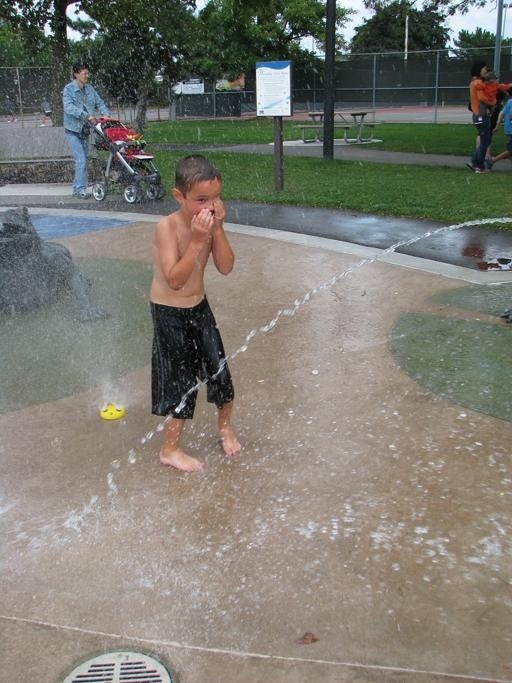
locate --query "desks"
[308,111,368,143]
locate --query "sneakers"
[475,120,483,124]
[466,155,493,173]
[73,188,91,199]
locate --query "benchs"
[334,121,381,143]
[297,124,355,144]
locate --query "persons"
[40,97,55,127]
[148,154,243,475]
[465,59,512,174]
[62,63,112,200]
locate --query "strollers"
[92,116,165,204]
